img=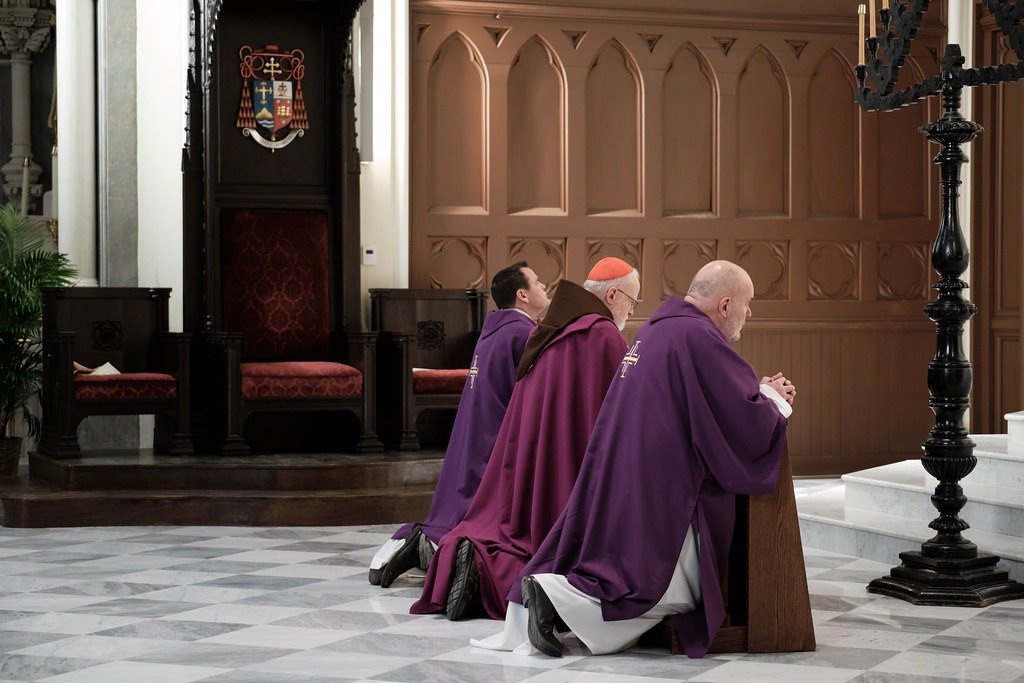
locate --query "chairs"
[37,287,196,459]
[369,288,491,451]
[180,0,384,455]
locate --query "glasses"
[607,288,639,309]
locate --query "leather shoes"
[381,527,421,588]
[521,575,564,656]
[369,565,386,585]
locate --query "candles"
[21,155,30,217]
[858,4,865,66]
[52,144,58,221]
[882,0,889,10]
[869,0,876,38]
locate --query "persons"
[469,259,797,659]
[369,261,551,589]
[409,256,641,621]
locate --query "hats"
[588,256,633,280]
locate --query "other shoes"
[447,540,480,621]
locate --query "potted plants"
[0,203,80,477]
